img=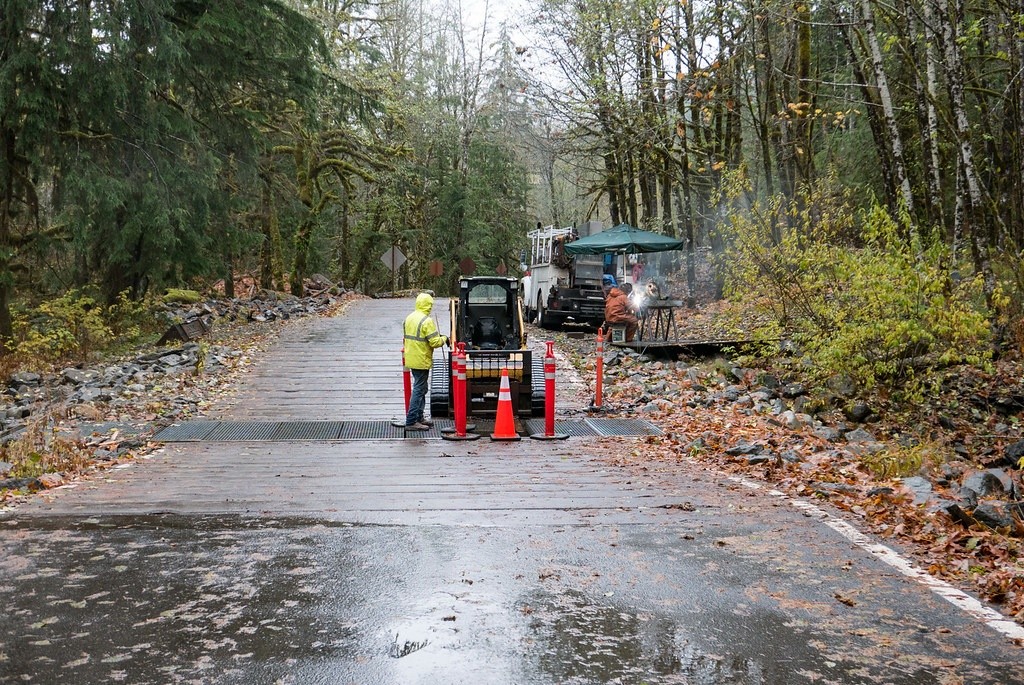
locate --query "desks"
[639,299,682,341]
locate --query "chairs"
[472,316,503,346]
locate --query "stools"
[609,325,626,343]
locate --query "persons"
[605,282,640,342]
[403,293,449,431]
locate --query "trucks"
[518,221,639,330]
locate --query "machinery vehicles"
[428,275,546,420]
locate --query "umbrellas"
[564,222,684,284]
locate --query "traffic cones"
[487,367,521,442]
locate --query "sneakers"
[405,422,430,431]
[420,418,434,426]
